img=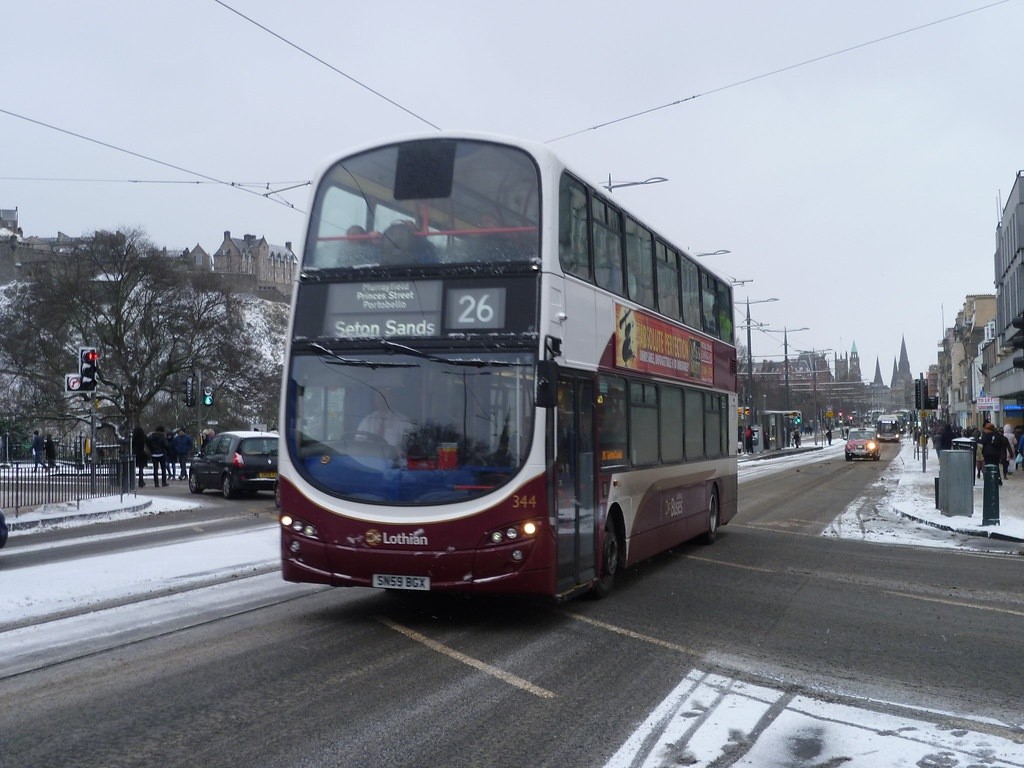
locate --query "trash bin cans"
[121,455,135,492]
[952,437,978,486]
[769,438,776,450]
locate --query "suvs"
[843,427,882,461]
[188,429,279,499]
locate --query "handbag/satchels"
[1015,453,1023,464]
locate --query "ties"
[378,417,385,437]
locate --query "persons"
[204,429,215,444]
[794,431,800,448]
[147,426,170,487]
[31,430,48,472]
[932,419,1024,485]
[477,212,497,237]
[827,429,832,445]
[174,428,193,481]
[746,425,755,453]
[719,306,730,342]
[44,433,57,471]
[338,221,438,264]
[132,427,150,487]
[166,430,176,481]
[355,386,413,458]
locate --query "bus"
[272,130,739,605]
[863,408,912,443]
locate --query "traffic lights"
[181,375,197,408]
[1011,316,1024,370]
[65,346,98,393]
[794,417,801,425]
[849,417,853,424]
[838,413,843,424]
[203,386,214,406]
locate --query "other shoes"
[179,476,182,480]
[155,485,159,487]
[163,484,169,487]
[999,478,1002,485]
[184,477,187,480]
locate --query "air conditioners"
[984,321,996,340]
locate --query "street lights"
[765,326,810,411]
[442,367,494,458]
[733,295,779,426]
[602,171,670,193]
[801,347,832,445]
